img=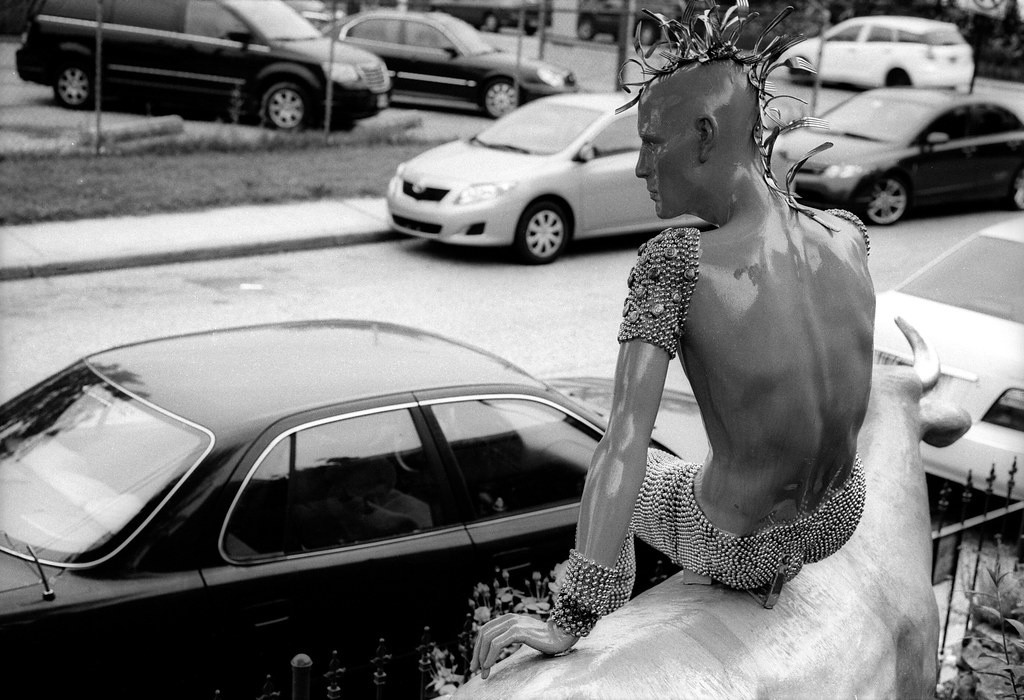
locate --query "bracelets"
[550,549,618,637]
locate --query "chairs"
[341,454,431,541]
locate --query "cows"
[433,314,975,699]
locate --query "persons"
[467,0,876,678]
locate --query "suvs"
[14,0,392,133]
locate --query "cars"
[384,93,782,266]
[0,319,712,699]
[278,0,348,31]
[577,1,696,47]
[410,0,554,36]
[320,7,580,120]
[867,217,1023,501]
[780,86,1024,228]
[776,15,978,92]
